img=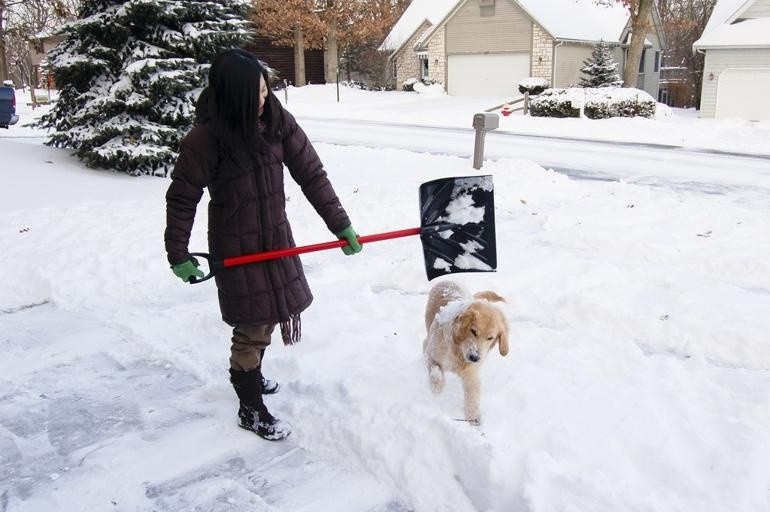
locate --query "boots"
[229,347,291,441]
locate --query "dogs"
[422,278,511,424]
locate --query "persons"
[165,49,365,441]
[0,80,16,129]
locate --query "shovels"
[180,173,498,283]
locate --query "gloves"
[335,223,361,255]
[173,258,205,282]
